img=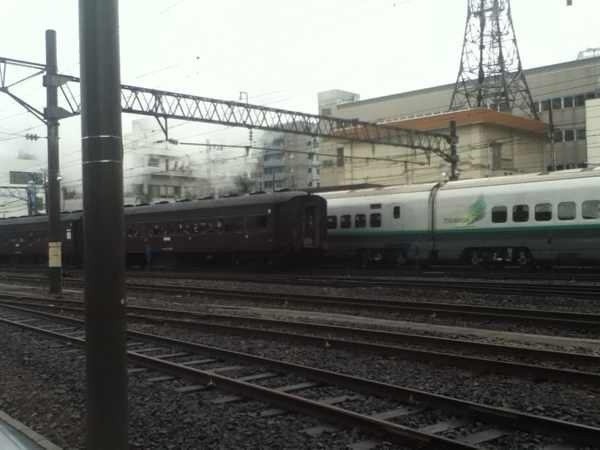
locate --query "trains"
[308,163,600,273]
[0,189,328,274]
